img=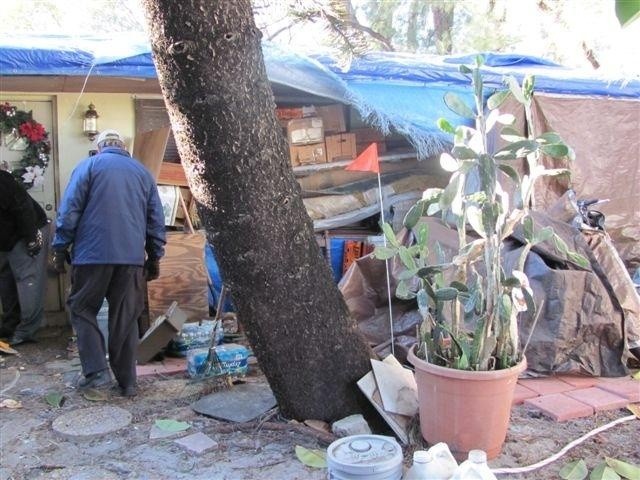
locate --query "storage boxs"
[278,103,358,166]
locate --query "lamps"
[84,103,101,140]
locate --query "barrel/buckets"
[446,448,499,480]
[405,442,459,479]
[326,433,405,480]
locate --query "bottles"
[175,321,248,379]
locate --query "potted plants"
[363,48,592,455]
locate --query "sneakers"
[113,380,138,397]
[7,332,32,345]
[79,368,113,391]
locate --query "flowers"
[0,102,52,187]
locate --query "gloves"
[27,230,44,257]
[146,257,160,281]
[53,248,72,274]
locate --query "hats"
[97,128,124,144]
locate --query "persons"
[0,170,50,346]
[51,130,167,396]
